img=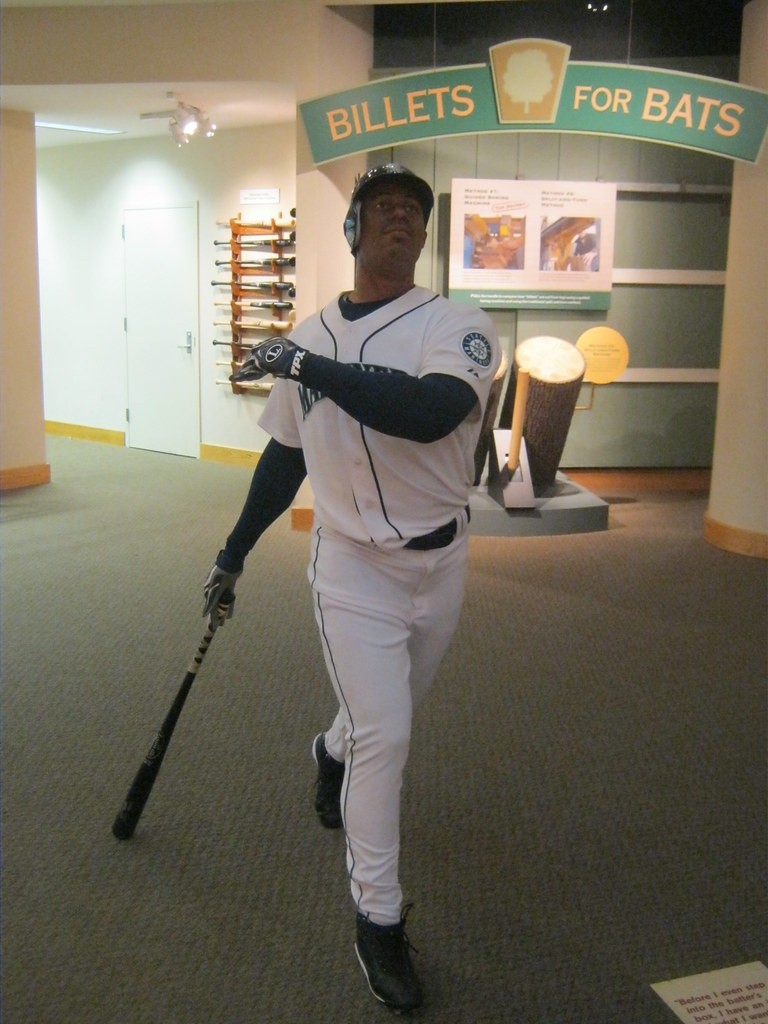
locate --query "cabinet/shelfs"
[230,212,283,394]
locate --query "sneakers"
[311,731,345,829]
[354,902,424,1010]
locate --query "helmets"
[344,161,434,250]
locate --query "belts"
[372,503,470,551]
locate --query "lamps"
[169,100,217,147]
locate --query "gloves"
[228,336,310,383]
[202,549,243,632]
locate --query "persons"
[202,164,502,1009]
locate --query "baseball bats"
[110,590,237,842]
[209,216,297,394]
